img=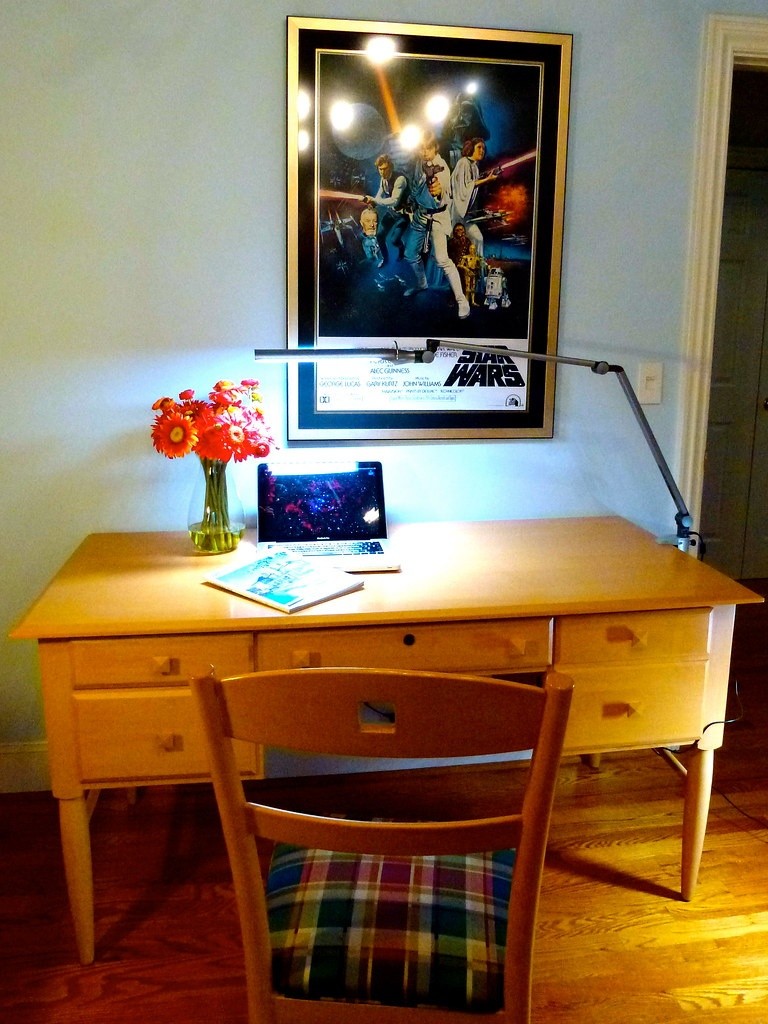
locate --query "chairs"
[185,669,575,1024]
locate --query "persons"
[352,90,508,319]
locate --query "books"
[202,546,365,613]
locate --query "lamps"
[247,337,700,555]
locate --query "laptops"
[256,461,401,572]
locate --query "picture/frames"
[285,15,573,442]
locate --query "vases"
[186,452,247,554]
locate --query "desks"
[9,511,765,965]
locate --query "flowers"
[144,378,275,552]
